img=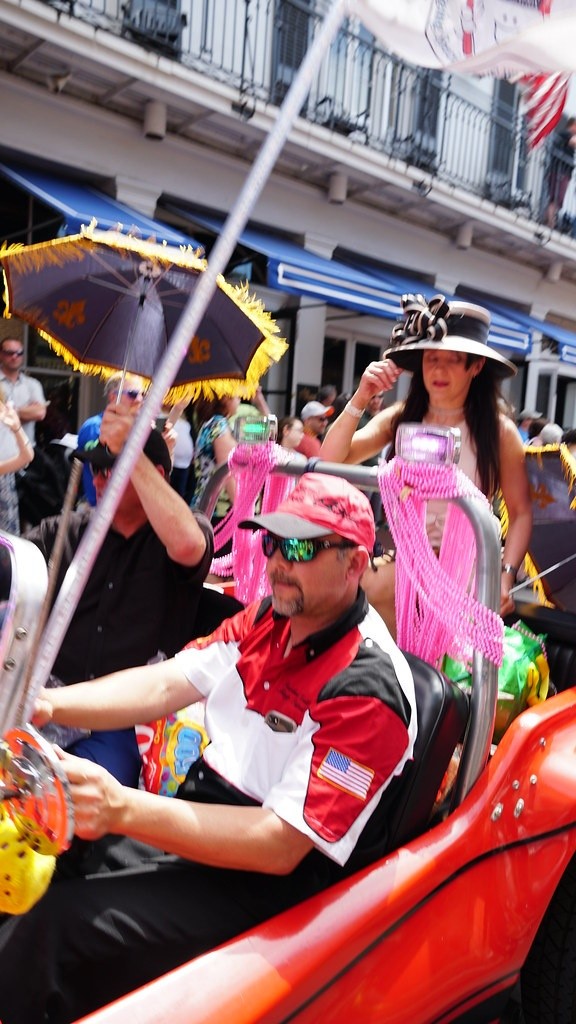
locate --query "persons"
[1,292,575,1024]
[540,116,576,232]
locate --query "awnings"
[167,202,534,356]
[2,163,204,257]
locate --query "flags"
[341,0,575,151]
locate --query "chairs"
[1,628,474,1011]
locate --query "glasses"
[91,462,113,479]
[2,348,23,356]
[262,534,356,562]
[115,390,146,400]
[371,393,384,399]
[319,416,328,422]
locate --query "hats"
[69,428,172,470]
[237,472,375,553]
[301,401,335,421]
[516,408,543,420]
[381,293,519,382]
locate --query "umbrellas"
[0,217,290,411]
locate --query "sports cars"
[0,460,576,1024]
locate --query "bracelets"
[11,425,23,433]
[500,561,519,576]
[343,400,366,418]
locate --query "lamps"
[457,223,474,250]
[143,100,167,142]
[327,174,347,205]
